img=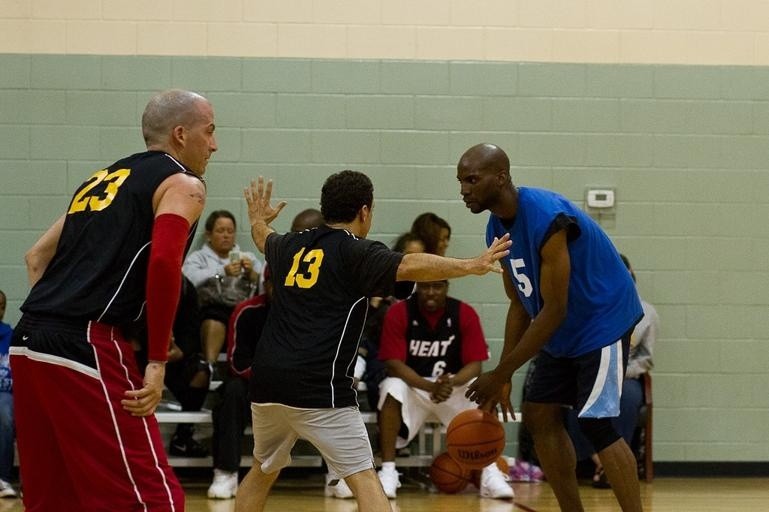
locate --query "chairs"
[638,372,654,485]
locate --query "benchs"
[14,353,522,493]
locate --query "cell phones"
[229,251,239,262]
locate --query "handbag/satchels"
[198,274,259,307]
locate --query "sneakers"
[326,471,354,499]
[168,430,206,458]
[480,471,514,498]
[206,468,240,500]
[376,470,402,500]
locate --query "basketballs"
[432,452,471,493]
[473,456,509,489]
[446,408,505,469]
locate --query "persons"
[182,210,264,398]
[130,273,212,457]
[9,86,217,512]
[1,291,27,501]
[589,253,658,489]
[207,170,516,499]
[456,142,647,512]
